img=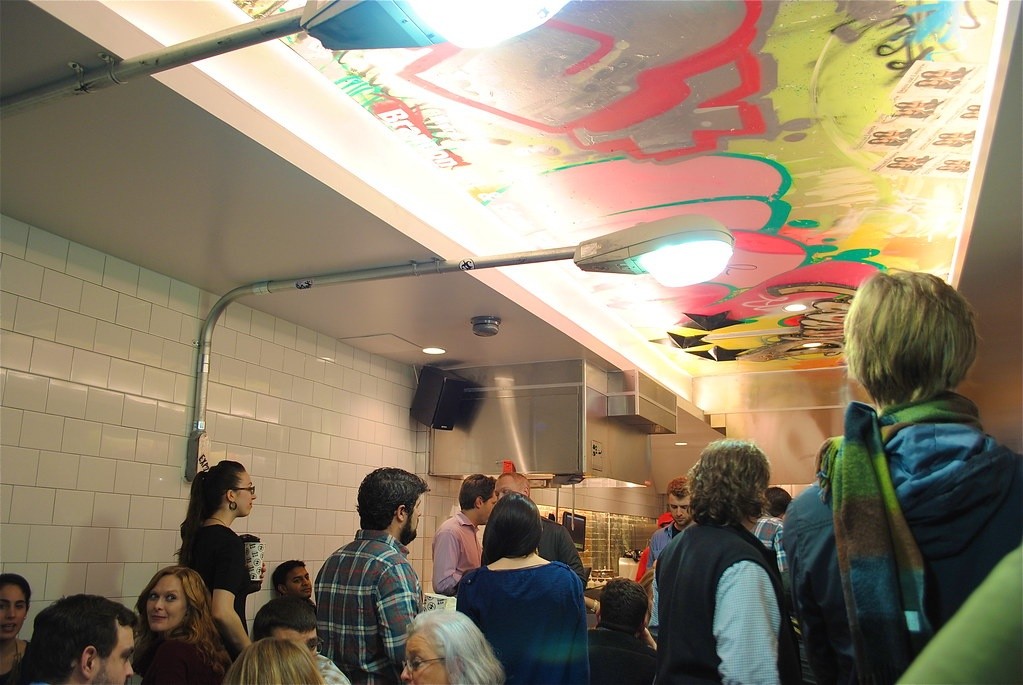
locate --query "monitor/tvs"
[562,511,586,552]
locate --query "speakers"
[413,365,468,430]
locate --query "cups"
[245,542,266,582]
[422,593,447,613]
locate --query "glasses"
[307,637,325,654]
[222,485,255,494]
[401,657,445,672]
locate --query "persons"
[779,269,1023,685]
[0,440,794,685]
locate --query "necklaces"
[209,518,225,526]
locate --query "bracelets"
[589,600,599,614]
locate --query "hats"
[659,512,673,527]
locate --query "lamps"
[185,212,734,483]
[0,0,575,120]
